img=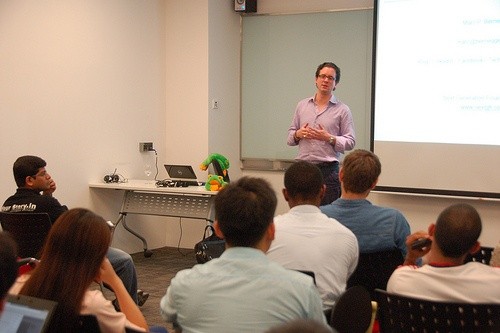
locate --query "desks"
[89,178,227,259]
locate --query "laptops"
[164,165,206,186]
[0,294,58,333]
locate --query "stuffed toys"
[200,152,231,176]
[205,175,229,192]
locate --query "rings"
[304,135,305,138]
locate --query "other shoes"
[112,289,149,314]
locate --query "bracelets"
[405,258,416,264]
[327,135,334,144]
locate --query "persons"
[159,175,334,333]
[0,231,18,315]
[287,61,355,205]
[386,203,499,305]
[16,208,169,333]
[318,149,423,267]
[265,161,359,326]
[1,155,150,312]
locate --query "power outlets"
[139,141,154,153]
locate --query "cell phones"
[411,238,432,250]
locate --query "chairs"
[466,246,494,266]
[348,248,406,302]
[44,309,101,333]
[376,290,500,333]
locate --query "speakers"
[234,0,257,13]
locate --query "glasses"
[318,74,336,81]
